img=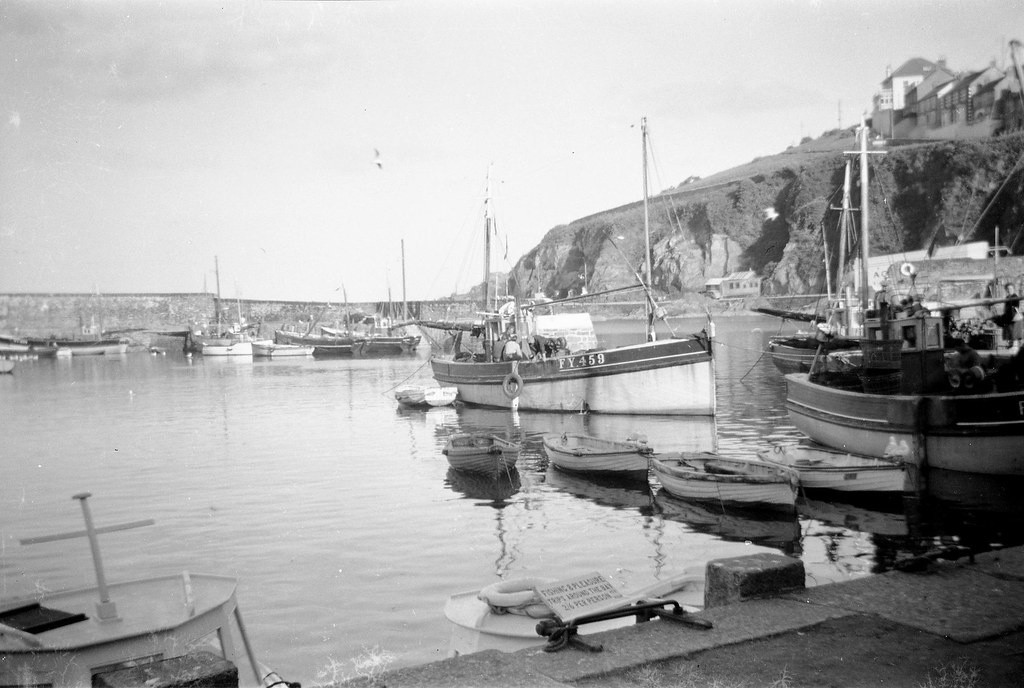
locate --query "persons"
[493,326,549,362]
[1002,282,1020,349]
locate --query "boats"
[783,289,1024,475]
[352,337,420,357]
[252,344,315,357]
[759,447,913,496]
[541,435,647,474]
[276,330,352,355]
[200,339,274,355]
[433,327,715,415]
[395,385,459,408]
[648,454,798,514]
[771,335,865,376]
[443,434,518,480]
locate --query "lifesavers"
[478,577,559,607]
[249,331,256,338]
[503,373,523,399]
[856,312,864,325]
[901,263,914,275]
[506,602,554,618]
[270,348,274,352]
